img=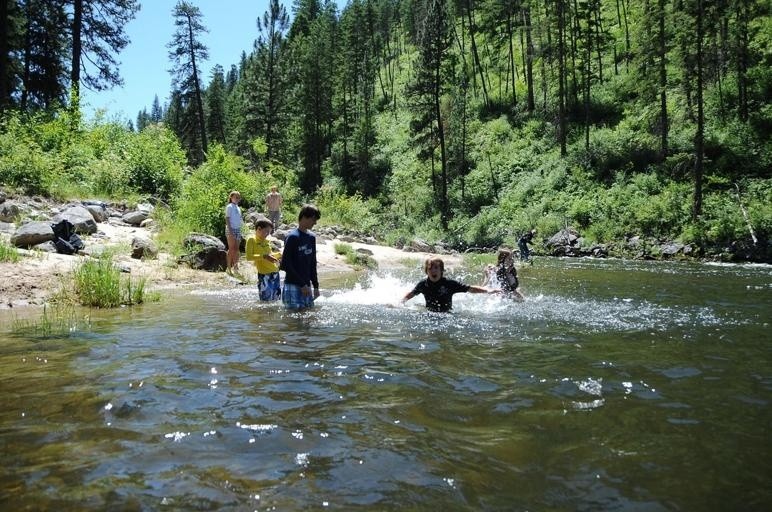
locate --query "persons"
[491,248,522,301]
[245,218,281,303]
[401,257,501,317]
[516,228,536,259]
[224,190,244,276]
[278,204,322,308]
[264,185,282,231]
